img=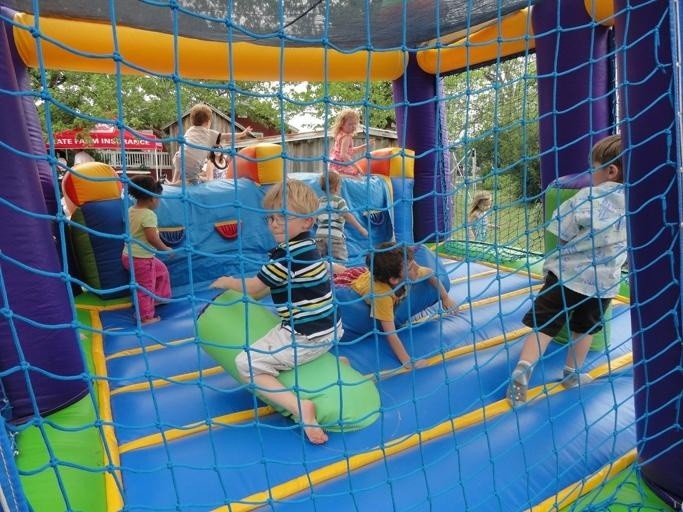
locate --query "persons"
[325,108,376,178]
[312,170,369,262]
[325,241,464,371]
[119,174,173,329]
[205,177,347,448]
[56,150,67,172]
[162,104,254,187]
[72,148,94,167]
[503,131,627,412]
[205,144,231,183]
[466,191,502,244]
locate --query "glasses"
[263,213,296,226]
